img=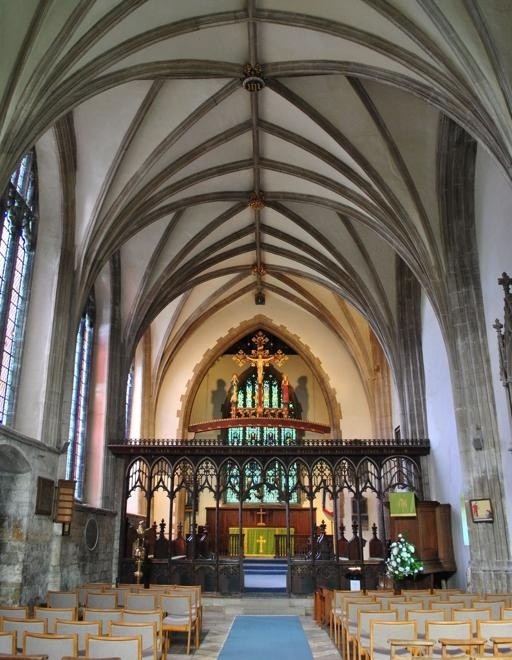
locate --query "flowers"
[384,528,424,580]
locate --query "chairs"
[469,498,494,523]
[0,582,202,660]
[314,587,512,660]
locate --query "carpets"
[216,614,314,660]
